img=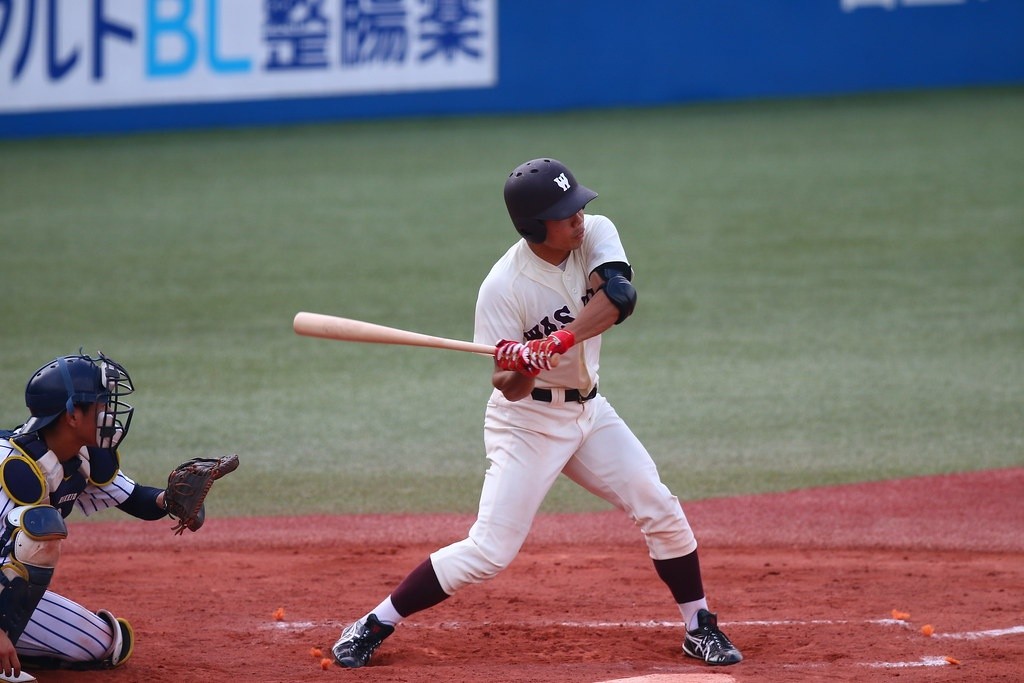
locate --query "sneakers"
[681,609,743,665]
[332,613,395,667]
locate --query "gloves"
[519,328,575,370]
[494,339,541,378]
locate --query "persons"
[0,355,168,683]
[332,158,744,669]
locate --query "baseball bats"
[289,309,563,372]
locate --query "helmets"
[504,158,599,244]
[12,357,110,434]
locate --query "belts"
[532,384,599,404]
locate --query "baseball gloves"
[165,454,241,537]
